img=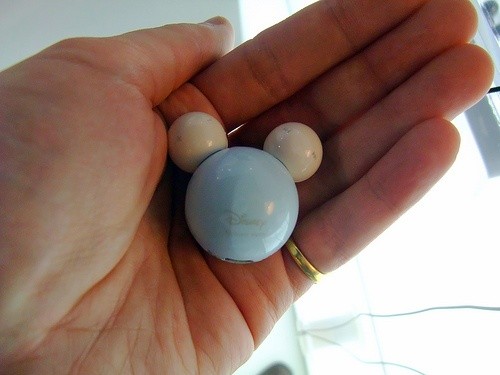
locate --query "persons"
[1,0,495,374]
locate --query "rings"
[285,238,326,284]
